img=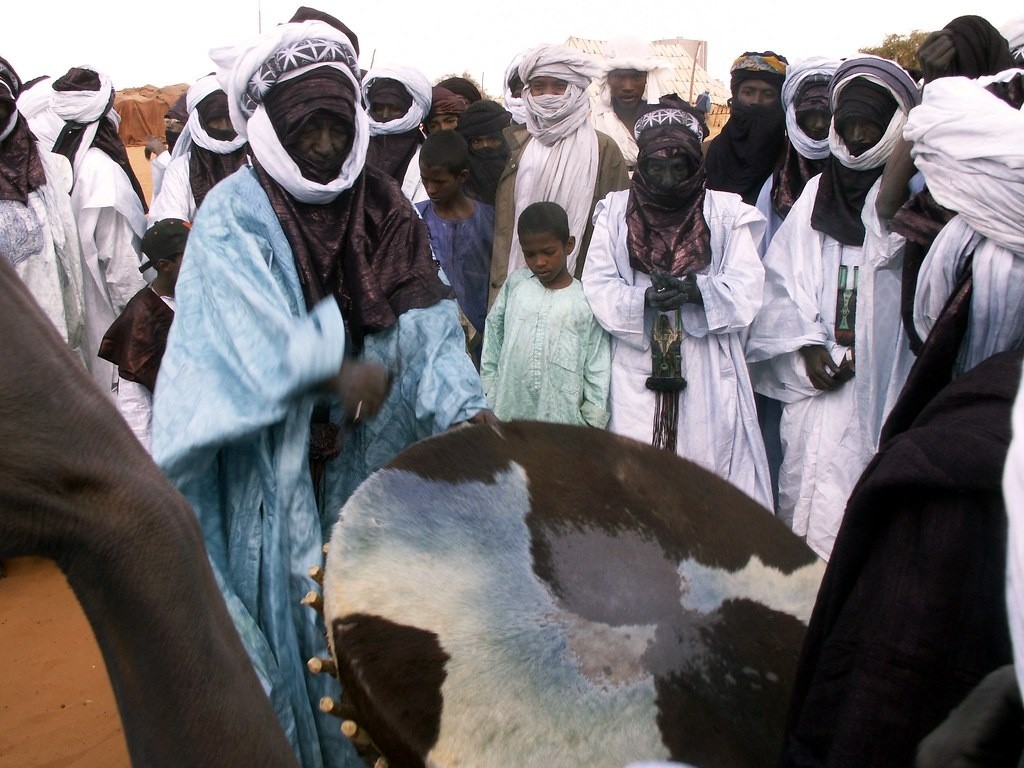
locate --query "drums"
[305,407,828,768]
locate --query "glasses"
[162,118,181,126]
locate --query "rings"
[657,288,665,293]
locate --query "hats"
[436,77,482,104]
[630,103,705,212]
[518,43,592,144]
[0,56,24,104]
[941,15,1015,96]
[828,54,923,171]
[782,55,842,160]
[51,66,117,126]
[600,41,657,74]
[228,6,372,205]
[731,51,789,131]
[185,73,247,153]
[140,217,194,262]
[368,78,412,113]
[163,93,190,126]
[420,86,467,123]
[458,99,513,168]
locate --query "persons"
[97,218,193,452]
[745,48,919,560]
[780,15,1024,768]
[0,55,150,397]
[488,44,630,313]
[492,50,528,125]
[422,77,482,136]
[362,63,432,203]
[0,258,298,768]
[148,20,504,768]
[144,93,189,203]
[590,35,662,179]
[414,129,495,364]
[455,101,513,206]
[580,93,775,517]
[755,55,845,262]
[141,71,252,281]
[704,51,790,206]
[480,200,611,429]
